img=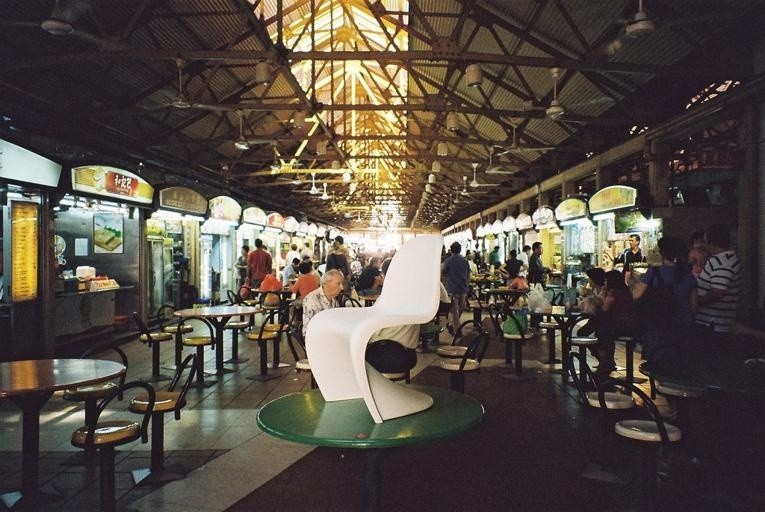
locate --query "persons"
[244,237,272,298]
[600,271,640,370]
[577,267,614,375]
[259,269,283,292]
[281,235,396,344]
[604,234,648,289]
[696,223,744,359]
[633,235,699,365]
[234,246,250,290]
[440,239,556,370]
[688,229,708,278]
[364,323,422,372]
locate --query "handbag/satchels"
[640,267,670,333]
[502,313,528,334]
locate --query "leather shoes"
[447,323,454,335]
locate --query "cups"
[62,269,74,279]
